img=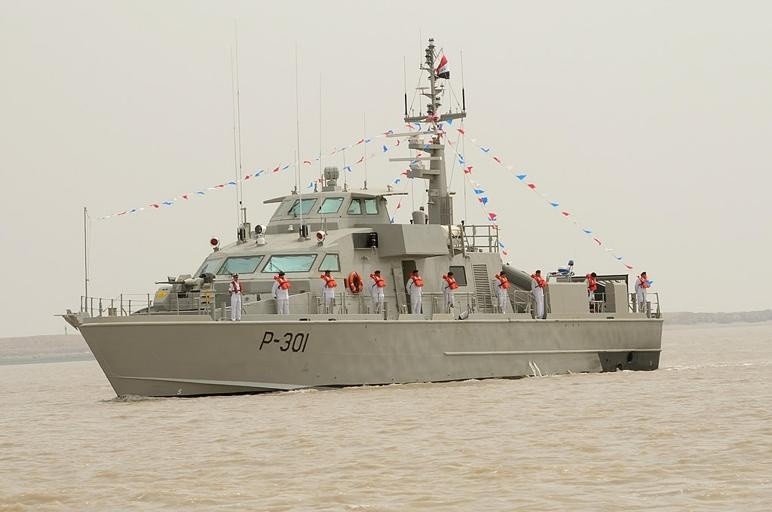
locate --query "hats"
[231,273,239,277]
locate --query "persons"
[272,271,289,314]
[406,270,422,314]
[321,270,335,314]
[368,271,384,313]
[531,270,544,318]
[442,272,455,313]
[229,273,242,320]
[635,272,647,313]
[584,272,597,301]
[494,271,508,314]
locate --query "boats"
[54,32,664,404]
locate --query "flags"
[433,48,450,80]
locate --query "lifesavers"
[349,272,364,292]
